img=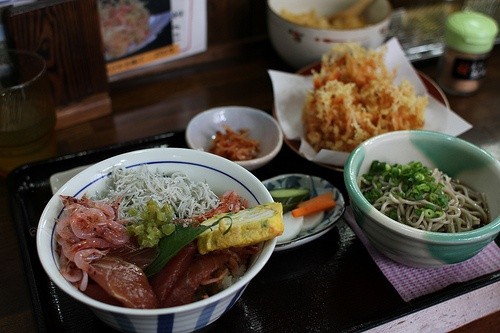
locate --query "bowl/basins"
[186,106,284,170]
[36,147,280,333]
[267,0,393,69]
[343,129,500,270]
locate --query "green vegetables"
[141,215,232,280]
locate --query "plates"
[260,173,346,252]
[104,11,172,61]
[273,60,450,174]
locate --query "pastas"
[361,168,488,233]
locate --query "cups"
[0,48,58,149]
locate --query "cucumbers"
[268,189,309,213]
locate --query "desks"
[0,52,500,333]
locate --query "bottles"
[439,9,498,97]
[0,6,23,89]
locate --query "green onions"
[360,160,449,221]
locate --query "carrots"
[292,192,337,217]
